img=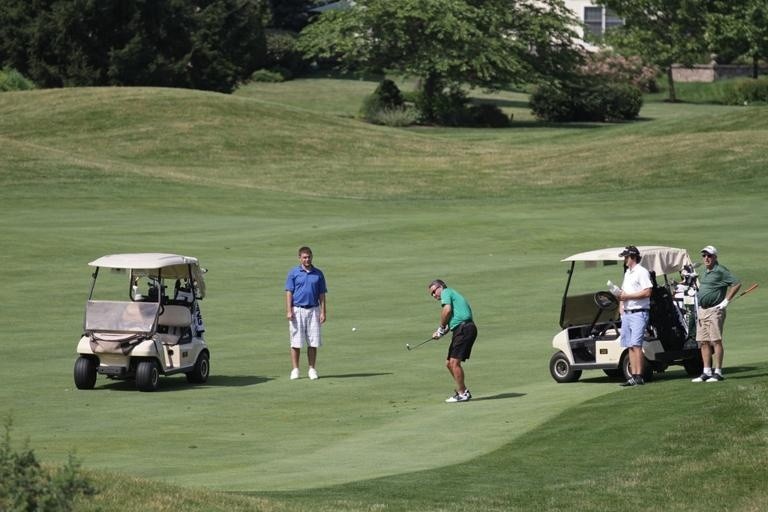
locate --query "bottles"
[607,280,623,298]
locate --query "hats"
[701,245,718,257]
[619,246,639,257]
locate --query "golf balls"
[352,328,356,331]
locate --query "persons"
[429,279,478,403]
[618,245,653,386]
[283,245,328,381]
[691,245,742,383]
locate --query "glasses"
[702,254,712,258]
[431,286,441,296]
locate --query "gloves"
[715,299,729,309]
[438,326,445,337]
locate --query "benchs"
[153,305,191,346]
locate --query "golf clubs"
[407,338,435,350]
[705,284,758,319]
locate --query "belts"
[461,320,472,325]
[296,305,313,309]
[624,309,648,313]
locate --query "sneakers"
[706,373,724,382]
[309,368,317,379]
[445,388,471,403]
[290,368,300,380]
[619,377,645,386]
[691,373,712,382]
